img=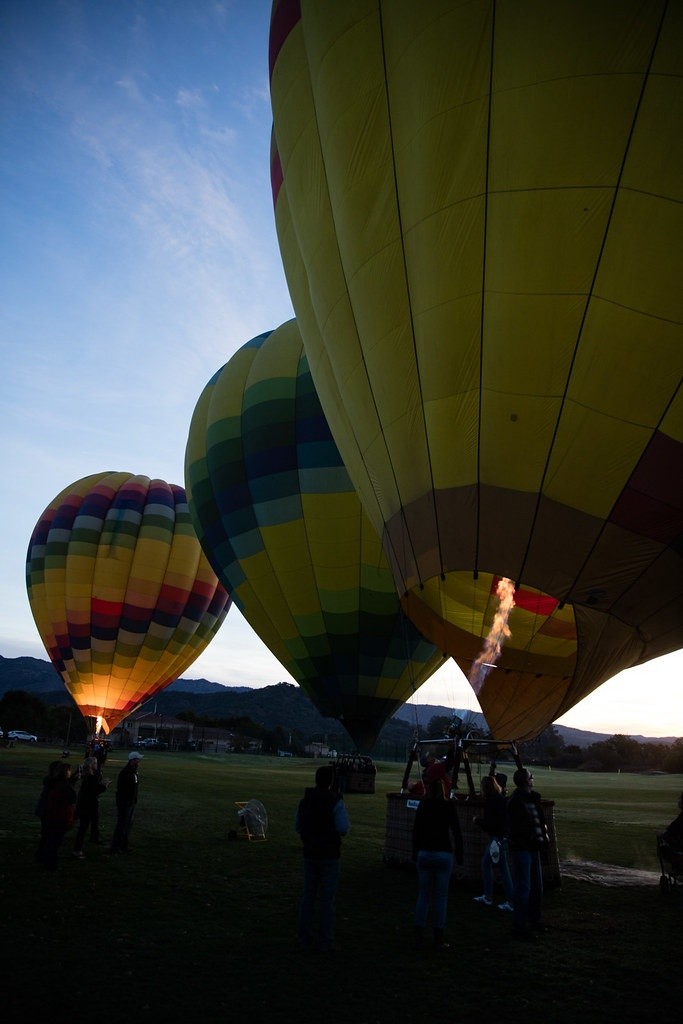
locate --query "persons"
[508,768,551,944]
[497,774,508,796]
[34,760,77,871]
[473,777,514,912]
[76,757,109,863]
[294,767,349,952]
[112,752,145,856]
[665,791,683,849]
[84,741,105,768]
[420,748,454,798]
[411,779,462,938]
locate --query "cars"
[133,739,168,748]
[0,727,4,738]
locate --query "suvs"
[8,731,38,743]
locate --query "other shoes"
[473,894,493,905]
[511,928,537,941]
[321,943,341,952]
[528,920,550,932]
[110,846,133,857]
[497,901,513,910]
[70,852,86,860]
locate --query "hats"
[128,750,143,762]
[482,777,493,784]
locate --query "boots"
[433,926,450,951]
[413,924,427,945]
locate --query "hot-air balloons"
[185,319,452,794]
[26,471,233,760]
[269,0,683,878]
